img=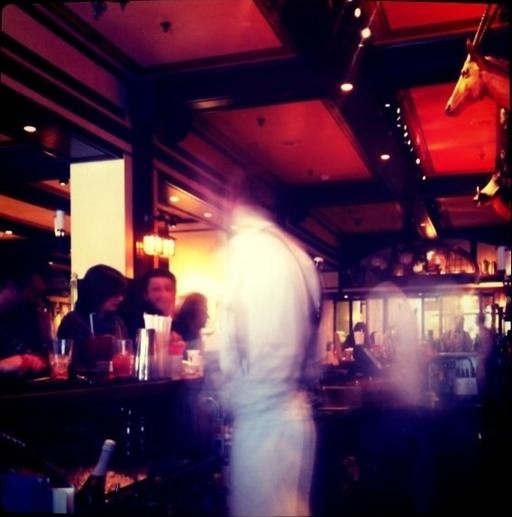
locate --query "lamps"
[143,228,177,256]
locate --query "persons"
[56,264,209,362]
[424,312,493,381]
[217,207,322,517]
[343,322,375,381]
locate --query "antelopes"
[445,2,512,116]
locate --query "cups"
[111,339,135,378]
[47,337,72,381]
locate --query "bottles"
[72,436,117,511]
[135,328,156,381]
